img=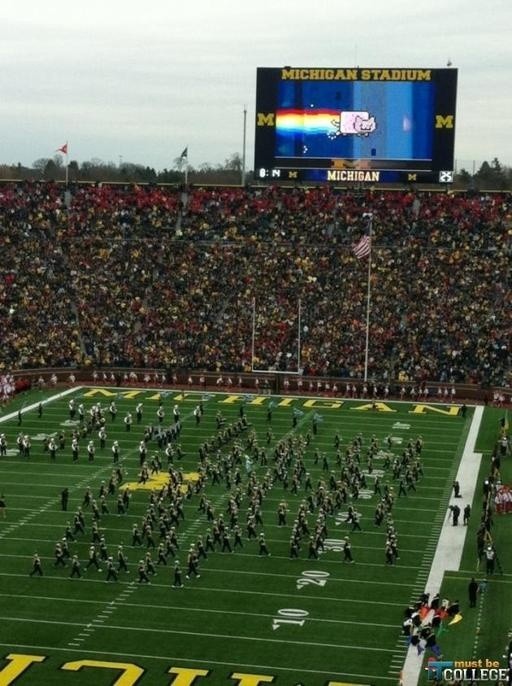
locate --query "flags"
[53,143,66,156]
[172,149,187,171]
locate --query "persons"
[451,479,463,499]
[1,408,428,594]
[1,178,512,409]
[467,576,480,607]
[475,415,511,563]
[460,402,468,418]
[482,545,497,575]
[399,592,512,686]
[448,502,461,526]
[463,503,472,526]
